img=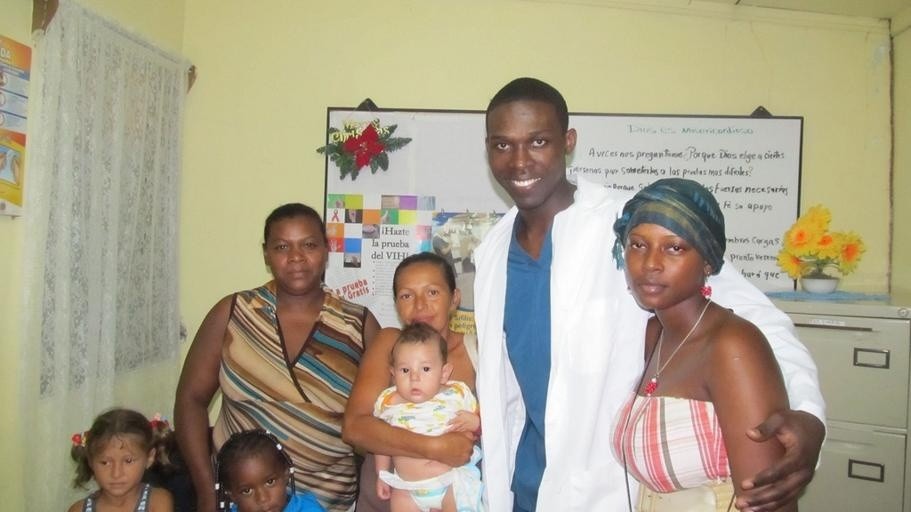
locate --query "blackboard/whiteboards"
[323,98,803,334]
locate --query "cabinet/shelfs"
[764,296,909,512]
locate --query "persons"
[213,427,327,512]
[611,176,798,511]
[372,317,483,512]
[173,201,383,512]
[339,249,483,512]
[469,76,830,510]
[64,408,178,511]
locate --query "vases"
[798,276,840,293]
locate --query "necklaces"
[643,298,715,398]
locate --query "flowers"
[318,117,412,181]
[773,203,865,280]
